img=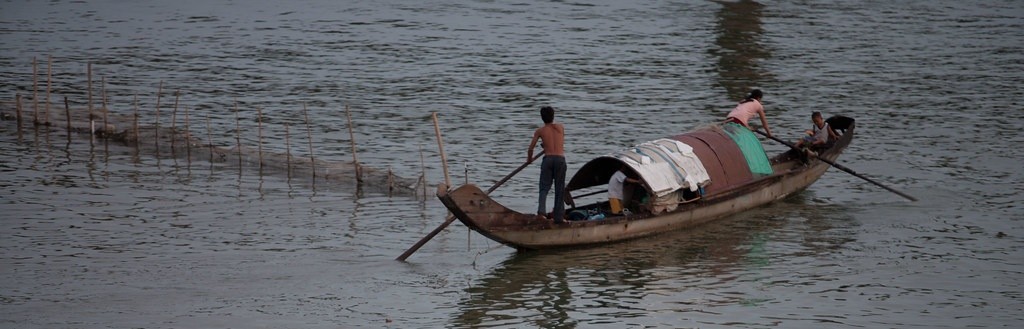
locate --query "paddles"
[754,128,919,202]
[395,147,546,263]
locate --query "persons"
[727,89,772,138]
[608,166,639,215]
[528,106,567,225]
[805,111,836,146]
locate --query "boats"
[436,113,854,250]
